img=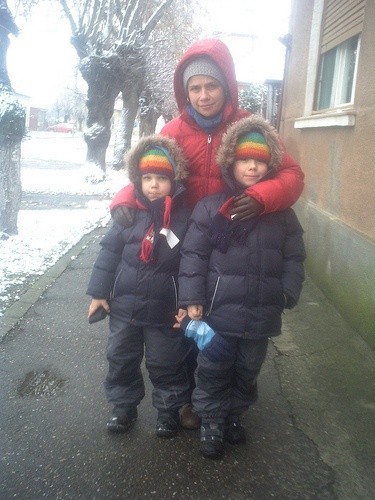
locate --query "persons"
[178,113,306,457]
[110,38,305,428]
[85,133,192,438]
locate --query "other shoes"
[178,401,203,430]
[199,421,224,457]
[107,404,138,431]
[156,411,178,435]
[224,414,246,443]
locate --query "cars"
[46,122,73,134]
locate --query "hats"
[137,148,173,176]
[235,130,271,162]
[181,57,229,98]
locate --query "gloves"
[113,206,137,226]
[228,191,264,222]
